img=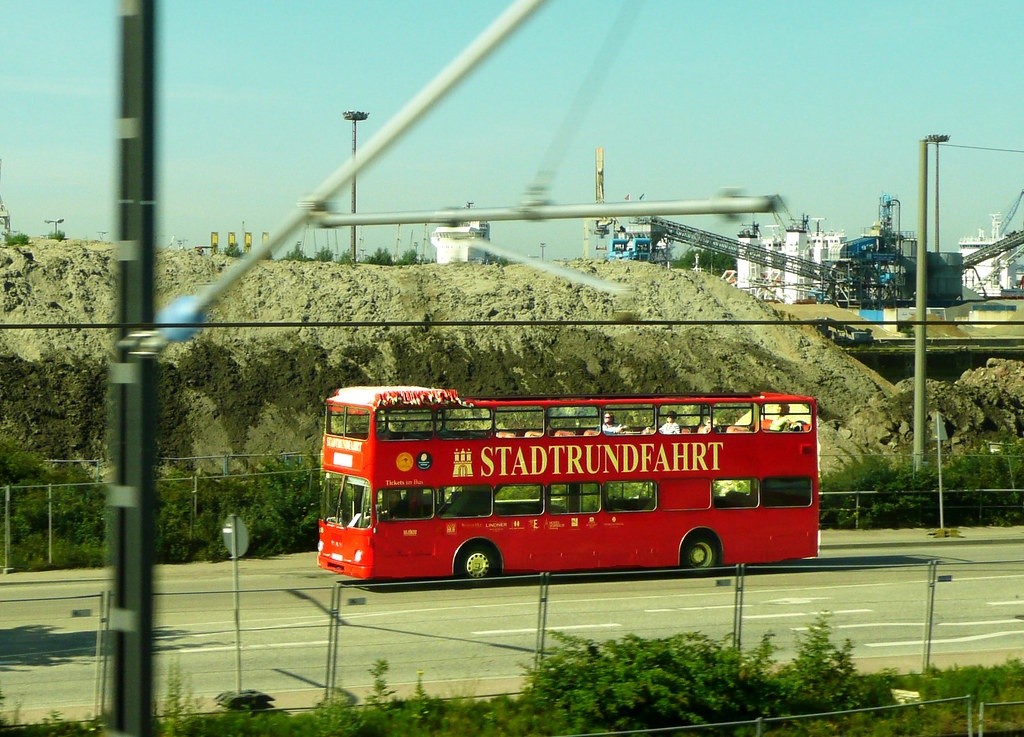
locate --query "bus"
[316,385,819,588]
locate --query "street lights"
[926,134,950,253]
[342,110,369,265]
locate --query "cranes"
[631,191,1024,309]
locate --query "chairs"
[495,419,811,438]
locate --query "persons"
[659,411,680,434]
[382,492,409,519]
[595,411,624,433]
